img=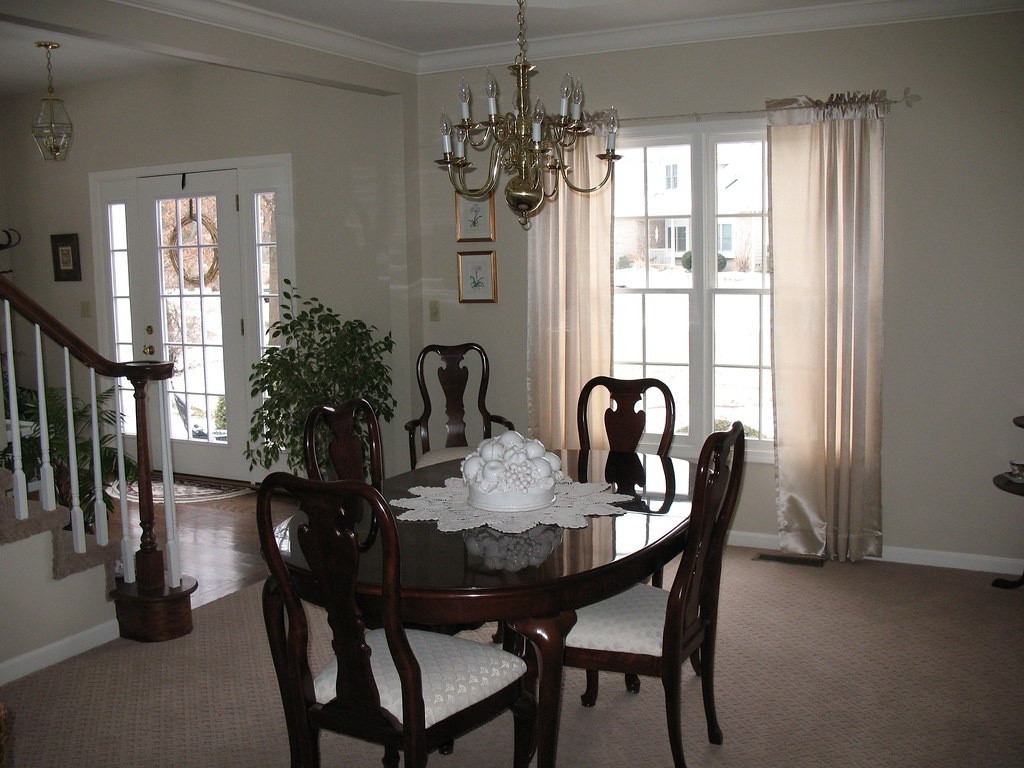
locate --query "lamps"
[434,0,624,228]
[30,42,75,162]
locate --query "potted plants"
[244,279,397,483]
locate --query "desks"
[260,450,728,768]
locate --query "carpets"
[105,471,258,504]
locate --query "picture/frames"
[454,189,496,243]
[456,251,499,305]
[49,233,81,281]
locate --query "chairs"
[404,343,515,470]
[577,376,675,590]
[255,469,541,768]
[304,398,503,644]
[502,421,747,768]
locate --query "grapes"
[505,462,533,494]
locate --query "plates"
[1004,474,1024,484]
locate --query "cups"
[1009,458,1024,479]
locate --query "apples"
[463,430,562,483]
